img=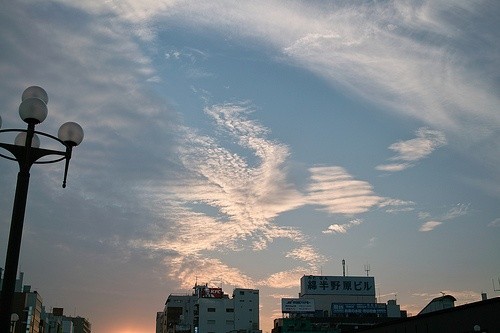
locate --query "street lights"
[0,86,84,333]
